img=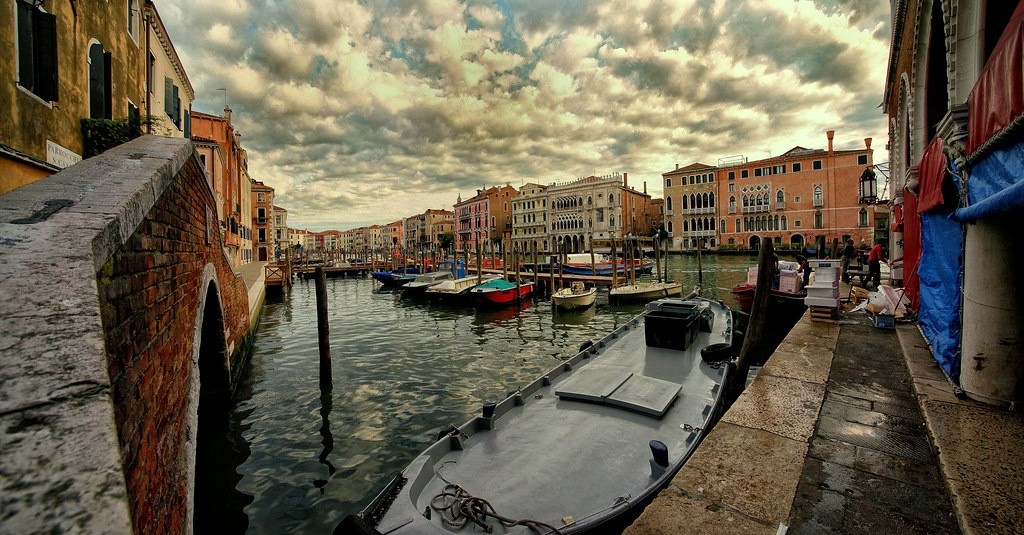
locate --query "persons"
[771,247,778,289]
[791,251,811,291]
[840,238,856,281]
[861,238,888,289]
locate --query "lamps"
[859,161,891,204]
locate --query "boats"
[332,297,736,535]
[370,260,467,286]
[469,276,534,303]
[608,274,682,300]
[551,281,598,309]
[550,251,654,277]
[423,272,506,301]
[401,272,456,295]
[732,261,810,315]
[466,259,511,268]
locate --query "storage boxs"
[643,299,712,351]
[748,260,896,329]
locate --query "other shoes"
[842,278,849,282]
[861,285,870,290]
